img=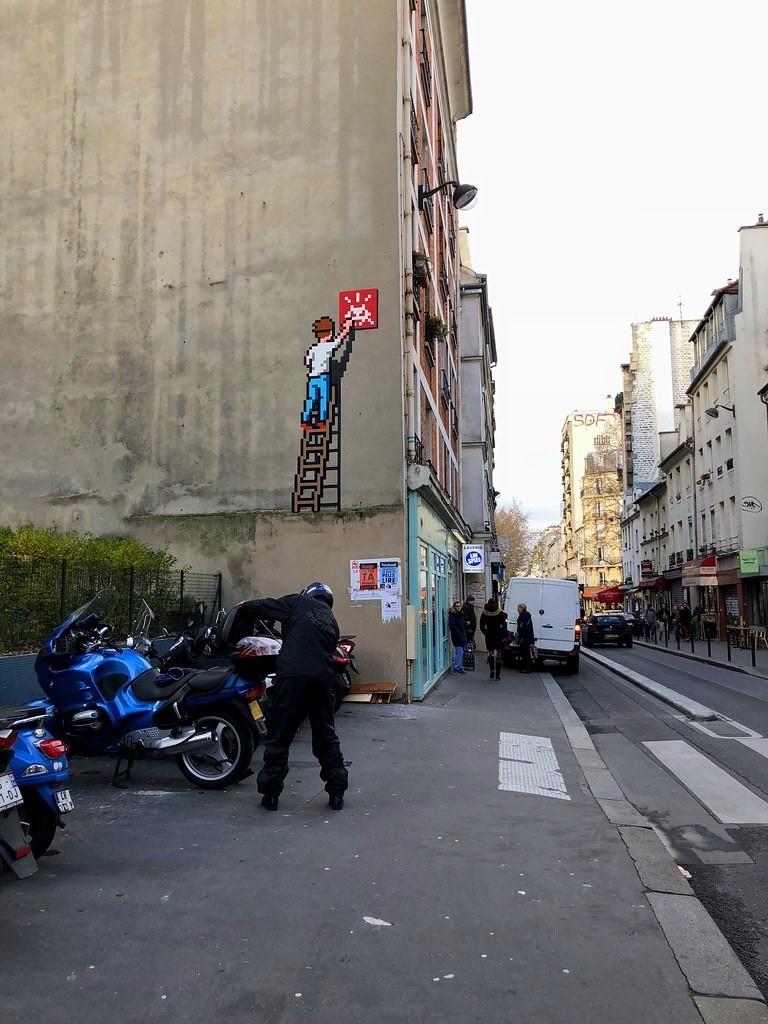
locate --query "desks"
[727,625,751,649]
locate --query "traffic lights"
[578,583,584,594]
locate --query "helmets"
[299,582,334,609]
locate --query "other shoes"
[495,675,501,681]
[453,669,466,675]
[519,669,532,673]
[328,794,345,810]
[489,672,495,680]
[261,795,279,811]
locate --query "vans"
[503,577,581,674]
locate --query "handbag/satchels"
[529,645,538,659]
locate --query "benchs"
[739,626,768,649]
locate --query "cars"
[623,613,642,636]
[582,613,632,647]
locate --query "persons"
[658,603,669,641]
[644,603,657,642]
[517,603,534,673]
[670,604,681,641]
[693,601,705,643]
[480,598,508,681]
[462,595,477,641]
[680,602,692,642]
[448,602,466,675]
[242,581,348,811]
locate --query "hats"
[466,594,475,601]
[488,598,496,603]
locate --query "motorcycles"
[0,706,74,858]
[125,599,360,751]
[24,589,264,789]
